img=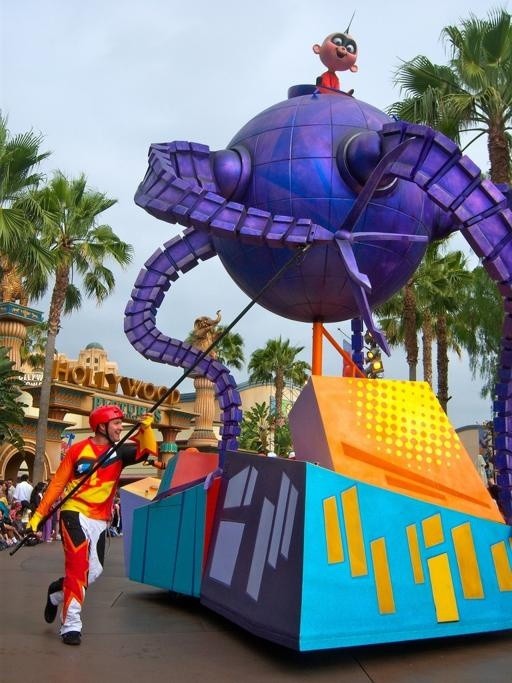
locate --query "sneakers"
[62,631,81,645]
[44,584,57,623]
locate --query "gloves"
[26,511,43,534]
[137,413,154,428]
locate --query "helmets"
[89,405,124,432]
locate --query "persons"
[1,472,122,549]
[23,405,160,646]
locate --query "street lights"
[486,420,497,485]
